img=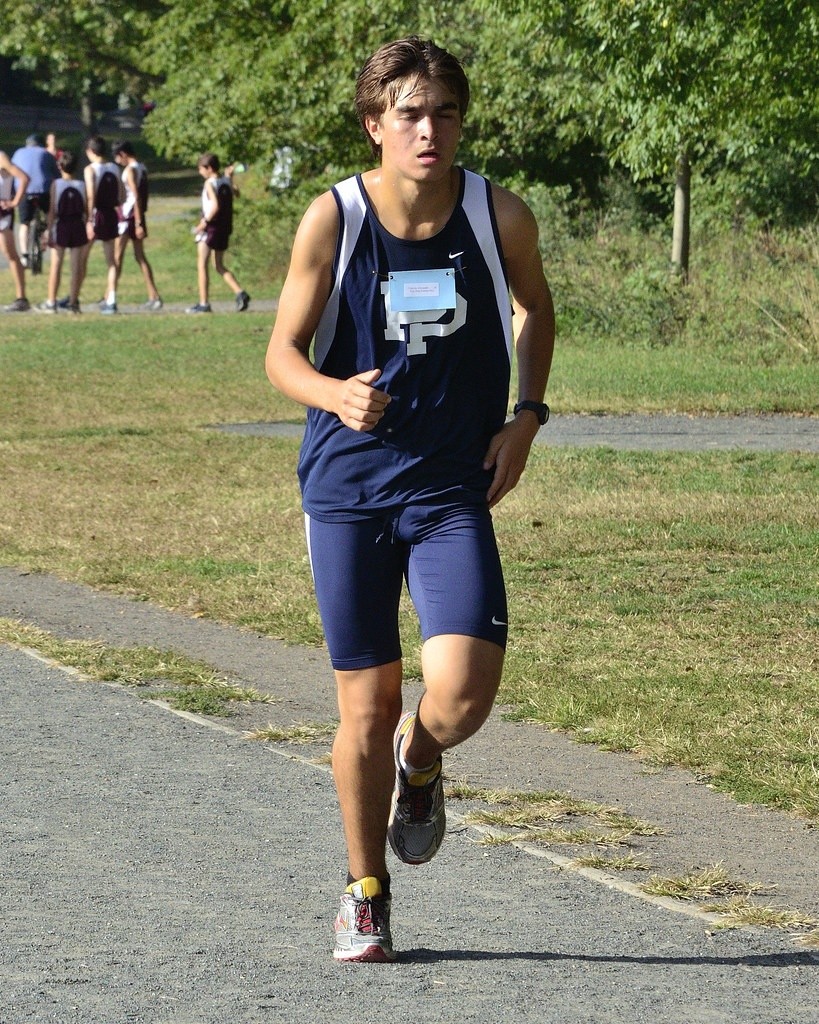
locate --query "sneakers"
[386,711,446,864]
[334,877,398,962]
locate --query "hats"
[26,134,48,147]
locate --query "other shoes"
[185,304,210,314]
[99,299,118,314]
[4,298,30,312]
[236,291,250,311]
[33,300,57,314]
[56,296,80,313]
[144,299,162,310]
[20,257,28,267]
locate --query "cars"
[106,102,153,135]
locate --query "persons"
[0,133,163,315]
[184,154,251,314]
[266,37,554,963]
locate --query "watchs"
[513,400,550,425]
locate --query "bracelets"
[203,217,210,226]
[87,219,93,222]
[135,222,142,227]
